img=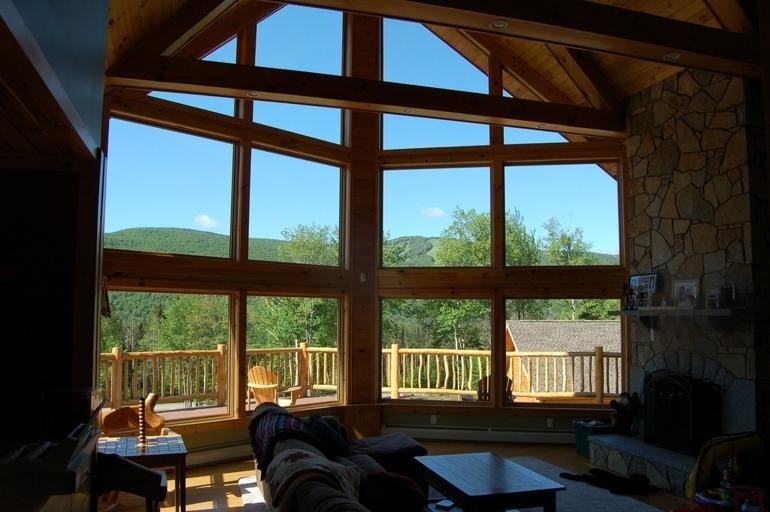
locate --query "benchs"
[587,433,696,496]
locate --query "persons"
[686,288,695,305]
[679,285,686,300]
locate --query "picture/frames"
[628,272,657,294]
[672,279,701,304]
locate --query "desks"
[89,434,188,511]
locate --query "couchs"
[248,402,428,512]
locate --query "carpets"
[417,455,665,511]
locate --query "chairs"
[477,372,515,401]
[89,450,168,511]
[246,365,302,411]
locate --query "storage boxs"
[572,417,616,457]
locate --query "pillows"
[312,416,349,449]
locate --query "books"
[435,499,455,511]
